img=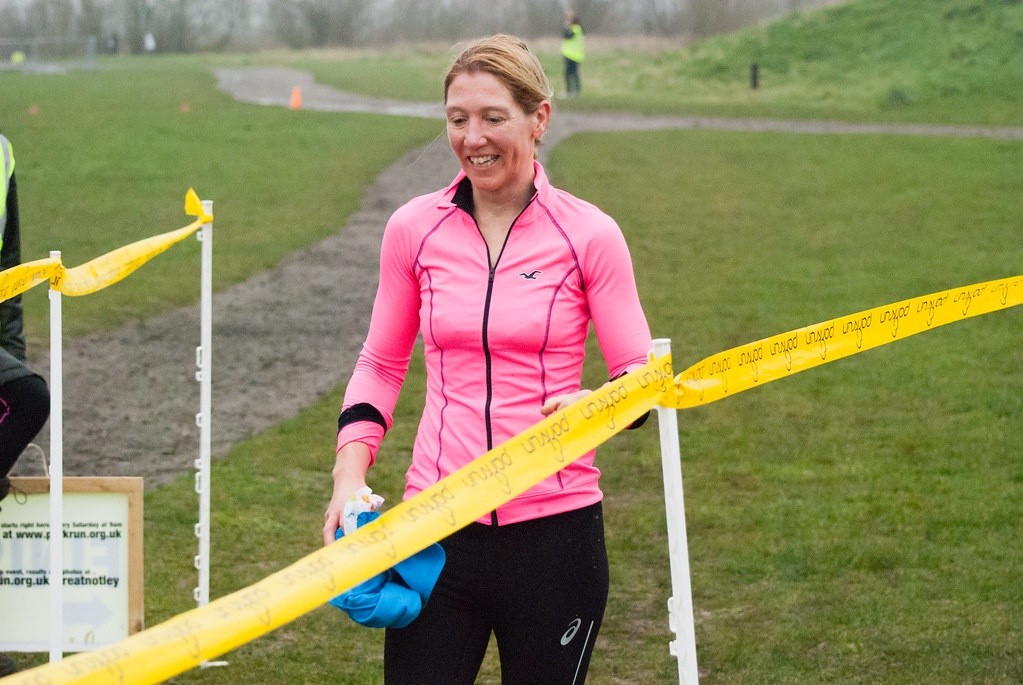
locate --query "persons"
[0,133,50,508]
[143,29,156,54]
[323,33,655,685]
[105,31,119,55]
[562,12,585,97]
